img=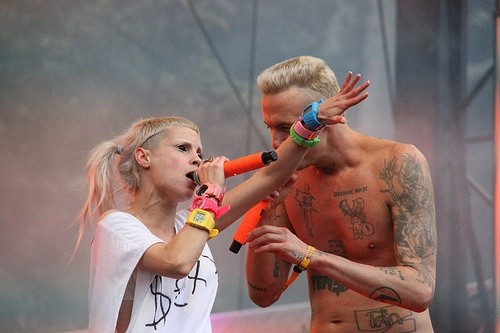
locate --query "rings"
[203,157,213,164]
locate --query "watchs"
[301,99,325,131]
[194,183,224,207]
[189,196,231,220]
[185,208,219,238]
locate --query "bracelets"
[290,116,321,148]
[274,245,315,301]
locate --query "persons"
[70,71,370,333]
[245,55,438,333]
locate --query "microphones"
[229,198,271,254]
[193,152,278,187]
